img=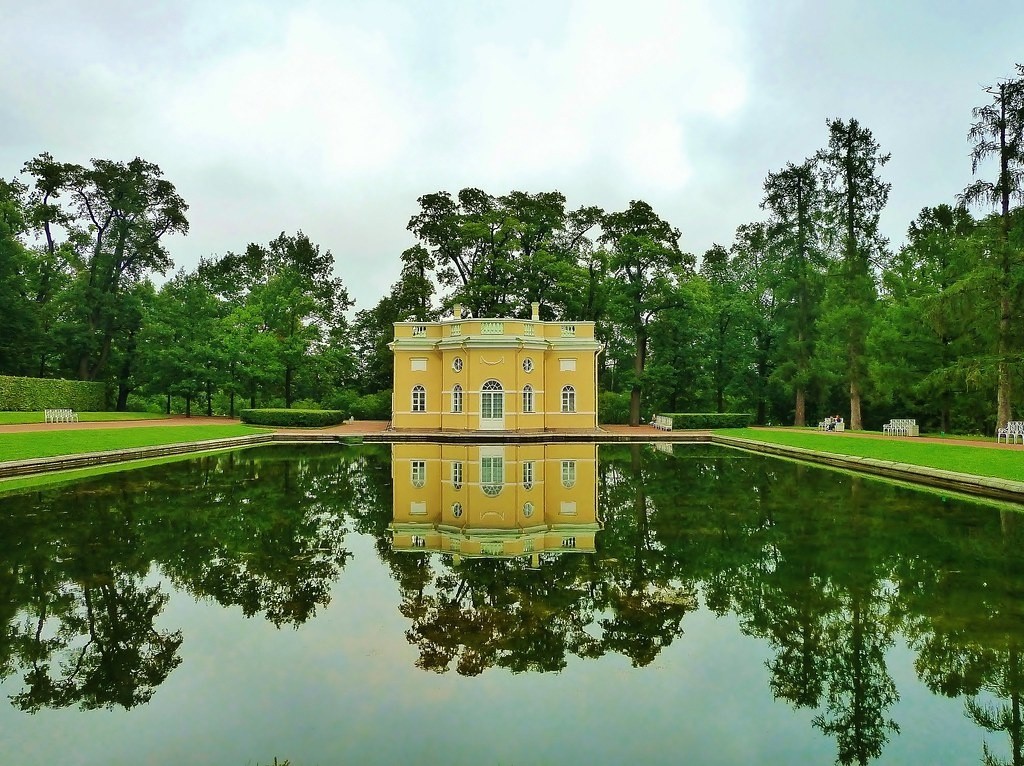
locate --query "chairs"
[997,421,1024,445]
[349,416,354,425]
[45,409,78,424]
[650,416,672,432]
[883,419,915,437]
[819,418,843,432]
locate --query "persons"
[829,415,842,431]
